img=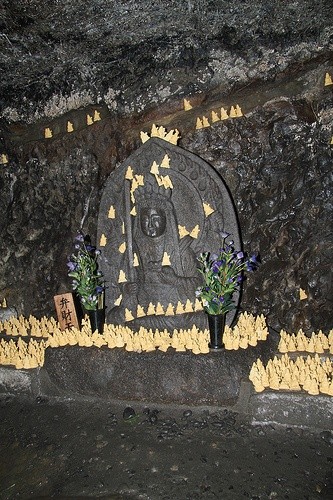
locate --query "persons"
[107,174,212,322]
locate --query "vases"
[204,311,228,351]
[86,306,107,335]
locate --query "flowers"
[194,231,258,315]
[66,228,108,310]
